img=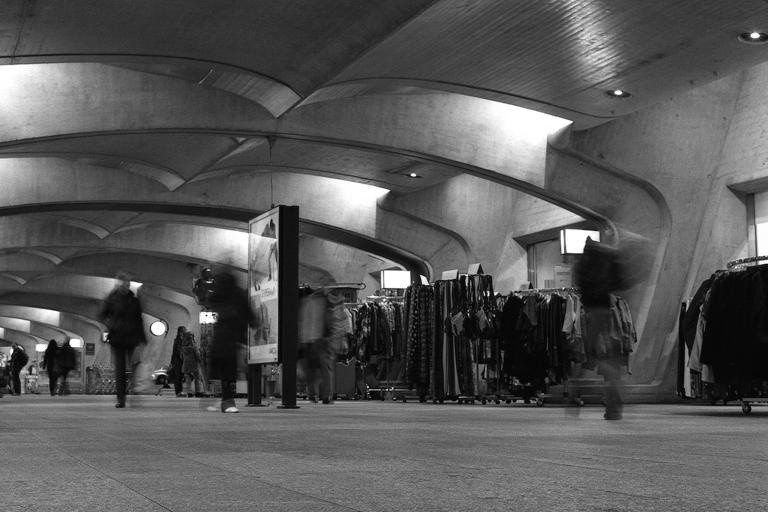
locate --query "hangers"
[726,256,768,273]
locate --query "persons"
[254,304,270,346]
[564,239,632,421]
[207,272,260,412]
[261,218,277,281]
[96,271,147,409]
[59,337,76,395]
[42,340,65,395]
[303,344,335,405]
[180,332,204,397]
[167,326,191,397]
[9,343,29,396]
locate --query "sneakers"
[225,406,239,413]
